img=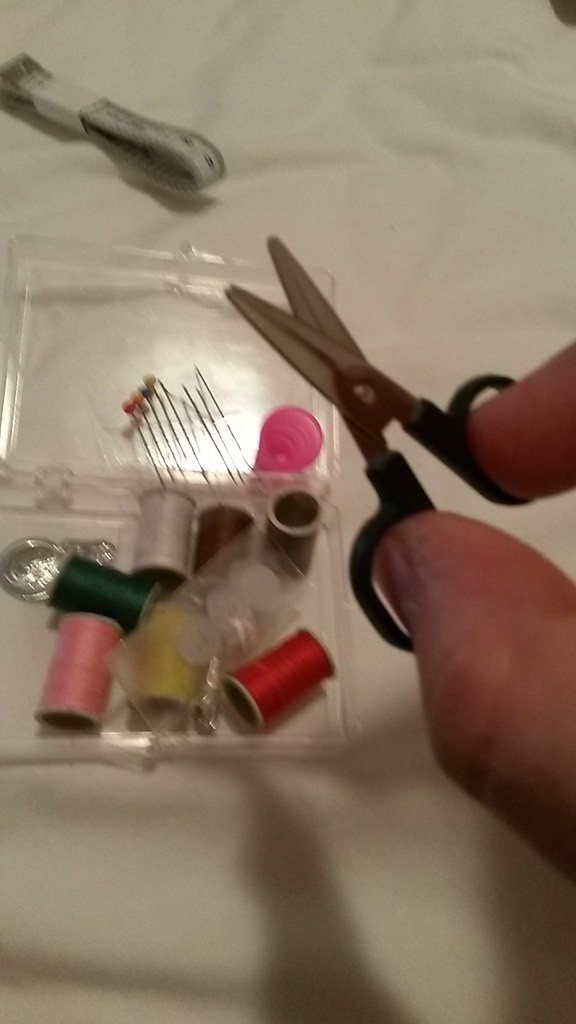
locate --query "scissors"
[224,234,541,653]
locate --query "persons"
[369,338,576,881]
